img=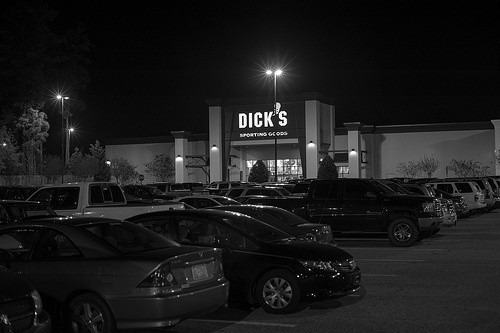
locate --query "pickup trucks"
[268,178,443,246]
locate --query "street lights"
[65,127,74,161]
[57,95,69,184]
[266,69,283,182]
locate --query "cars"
[203,205,337,248]
[124,209,360,313]
[383,176,500,226]
[0,216,230,333]
[0,178,312,223]
